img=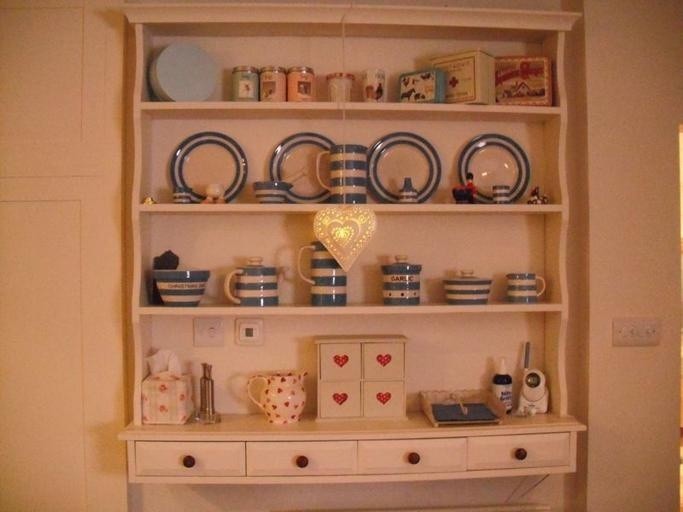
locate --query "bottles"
[286,66,315,102]
[259,65,288,102]
[399,177,419,204]
[231,65,260,102]
[492,356,514,413]
[378,254,422,306]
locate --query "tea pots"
[245,368,311,426]
[224,256,286,307]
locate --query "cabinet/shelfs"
[116,1,582,485]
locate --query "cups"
[505,271,547,303]
[296,241,352,306]
[315,144,368,205]
[326,72,355,102]
[492,185,511,205]
[171,186,192,204]
[360,68,389,102]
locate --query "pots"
[442,268,493,305]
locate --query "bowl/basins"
[253,181,288,204]
[151,267,211,307]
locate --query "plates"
[366,131,442,204]
[457,132,531,204]
[166,130,249,204]
[270,132,337,205]
